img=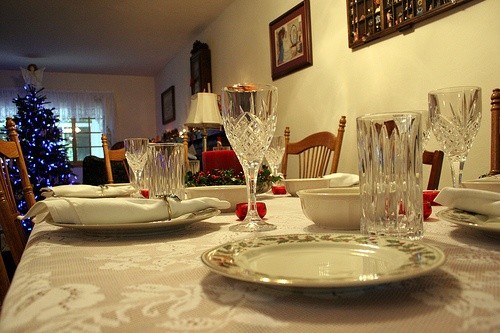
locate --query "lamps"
[184,82,223,152]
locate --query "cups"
[398,190,440,222]
[129,157,150,198]
[235,202,266,221]
[272,186,287,195]
[146,142,185,199]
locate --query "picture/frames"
[160,85,176,124]
[269,0,312,81]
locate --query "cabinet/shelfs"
[346,0,492,48]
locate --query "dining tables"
[1,184,500,333]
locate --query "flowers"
[185,170,282,188]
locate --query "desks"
[202,149,244,175]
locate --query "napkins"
[39,184,134,197]
[433,187,500,218]
[323,173,359,187]
[17,197,232,224]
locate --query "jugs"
[356,107,436,241]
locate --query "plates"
[436,205,500,239]
[50,208,221,236]
[201,230,445,291]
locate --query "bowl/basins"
[284,178,331,198]
[186,185,248,213]
[297,187,363,229]
[461,179,500,192]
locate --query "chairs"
[0,117,37,310]
[281,115,345,179]
[102,133,129,184]
[422,149,444,190]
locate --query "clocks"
[190,40,213,93]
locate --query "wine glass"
[124,138,149,198]
[220,82,277,232]
[265,136,285,185]
[428,86,482,188]
